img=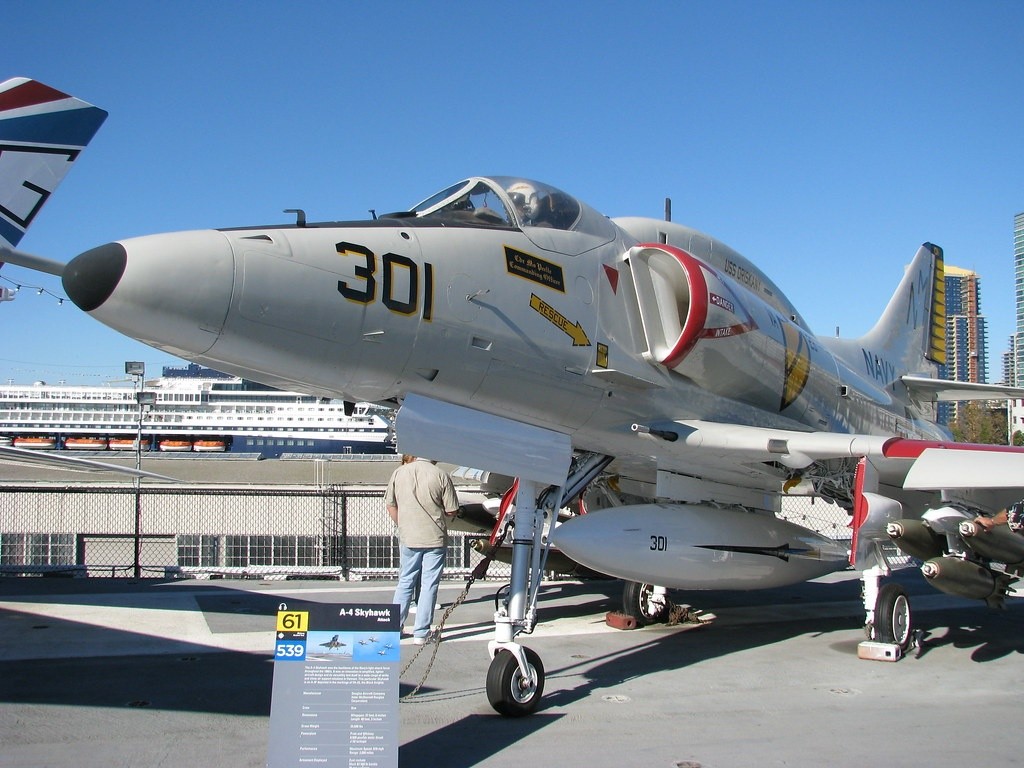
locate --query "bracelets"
[991,518,995,524]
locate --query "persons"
[974,498,1024,536]
[507,183,553,228]
[383,453,459,645]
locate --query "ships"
[0,361,421,464]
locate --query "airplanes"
[319,634,347,650]
[369,635,378,643]
[358,639,367,646]
[384,643,395,650]
[61,175,1024,721]
[378,649,389,657]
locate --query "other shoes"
[414,635,441,644]
[435,604,441,610]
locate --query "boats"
[159,438,194,452]
[108,436,150,451]
[14,434,57,450]
[64,435,108,450]
[0,435,13,446]
[193,440,226,452]
